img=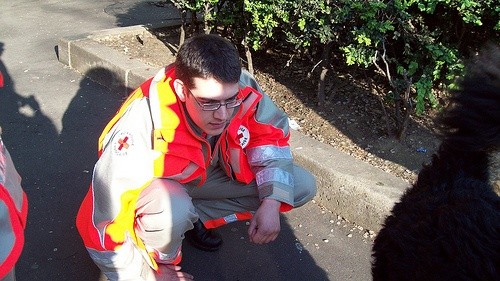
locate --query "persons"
[77,34,317,281]
[368,43,499,281]
[0,132,30,281]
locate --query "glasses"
[183,84,242,111]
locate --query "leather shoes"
[184,218,224,251]
